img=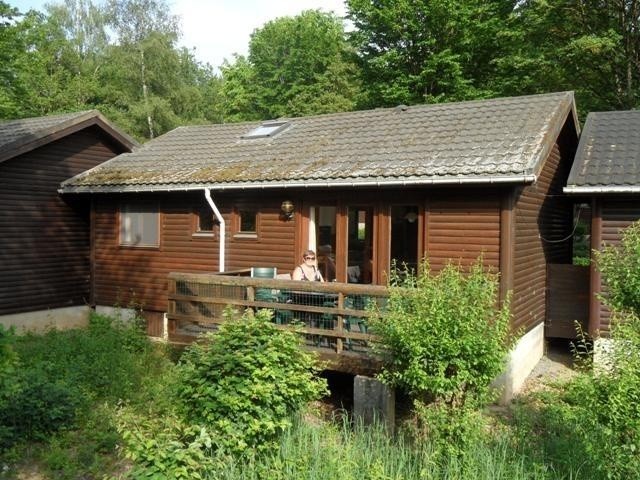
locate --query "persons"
[318,244,336,282]
[291,249,325,291]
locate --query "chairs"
[246,266,409,348]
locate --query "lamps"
[280,197,297,219]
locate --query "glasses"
[307,257,315,260]
